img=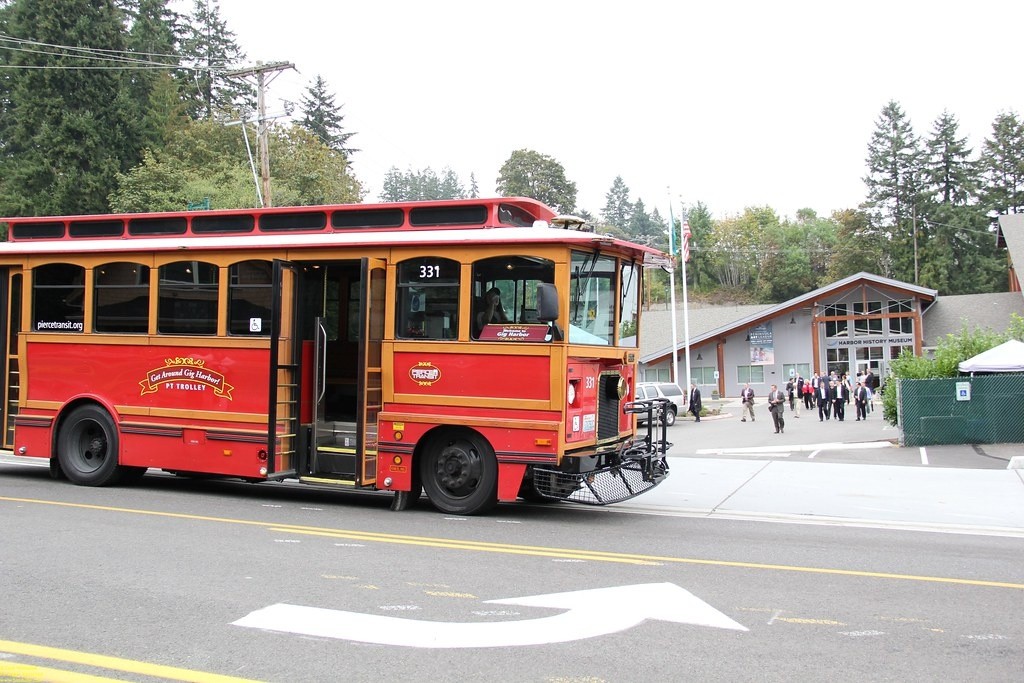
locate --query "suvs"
[633,382,686,427]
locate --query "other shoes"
[794,416,799,418]
[741,418,746,422]
[839,419,844,421]
[752,419,755,422]
[774,432,779,434]
[856,419,860,421]
[695,418,699,422]
[780,428,784,433]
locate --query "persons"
[741,383,756,422]
[786,368,876,422]
[477,287,508,331]
[768,384,786,434]
[880,377,891,420]
[853,382,867,421]
[689,383,702,422]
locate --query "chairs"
[472,296,484,339]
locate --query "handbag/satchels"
[769,405,773,412]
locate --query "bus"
[0,196,675,514]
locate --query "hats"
[483,287,500,297]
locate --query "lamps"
[697,354,703,360]
[790,312,796,324]
[745,331,751,341]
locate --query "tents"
[951,339,1024,443]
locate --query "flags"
[683,205,692,262]
[672,212,677,257]
